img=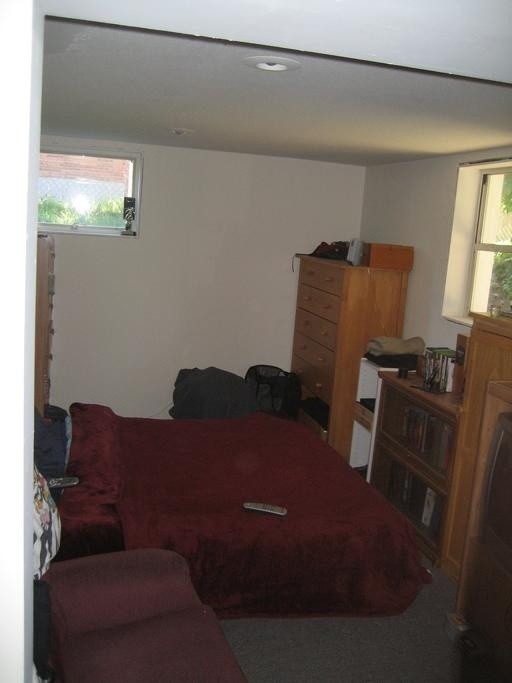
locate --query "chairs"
[34,467,246,682]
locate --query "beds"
[34,402,433,619]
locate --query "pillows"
[34,463,62,582]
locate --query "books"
[387,346,458,537]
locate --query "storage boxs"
[361,243,414,271]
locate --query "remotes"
[46,477,79,488]
[243,502,287,516]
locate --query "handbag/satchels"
[169,362,302,421]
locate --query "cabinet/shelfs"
[34,235,55,408]
[374,372,456,564]
[350,357,415,483]
[289,254,409,459]
[441,311,512,683]
